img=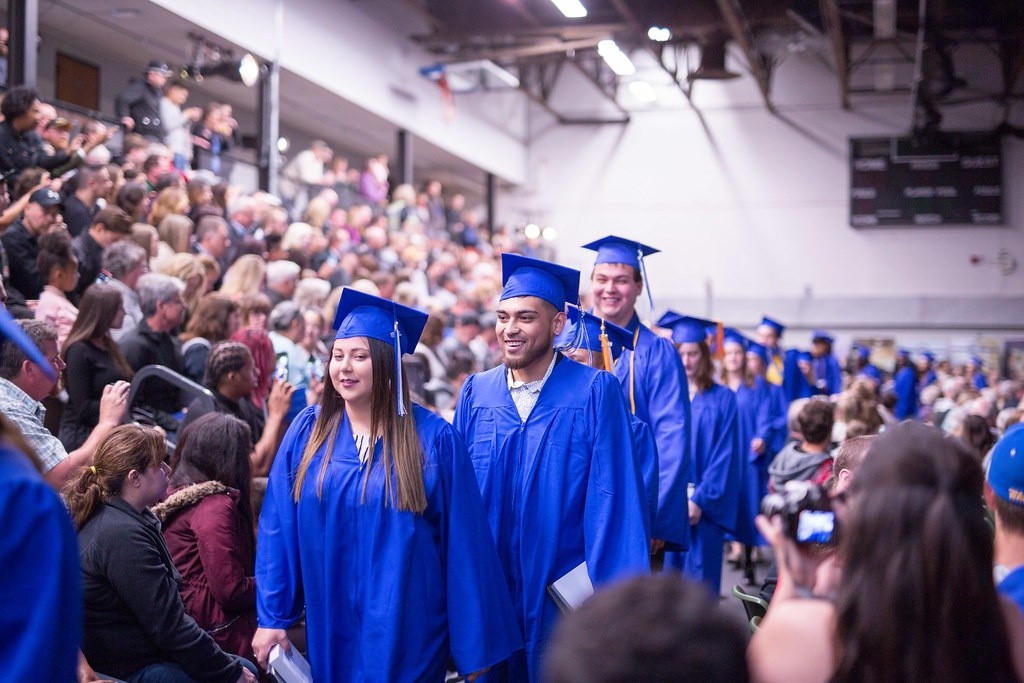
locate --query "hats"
[499,252,593,367]
[45,117,72,132]
[146,59,174,77]
[581,235,662,311]
[552,304,635,372]
[982,421,1024,506]
[0,302,56,380]
[658,310,983,377]
[332,287,429,416]
[30,188,66,212]
[453,311,481,326]
[477,312,498,328]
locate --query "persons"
[554,305,660,538]
[0,29,1024,683]
[582,235,689,558]
[452,253,654,683]
[657,311,742,600]
[251,287,524,683]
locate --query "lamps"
[216,54,260,85]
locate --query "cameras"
[788,506,840,546]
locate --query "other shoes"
[743,566,754,584]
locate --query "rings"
[253,654,256,657]
